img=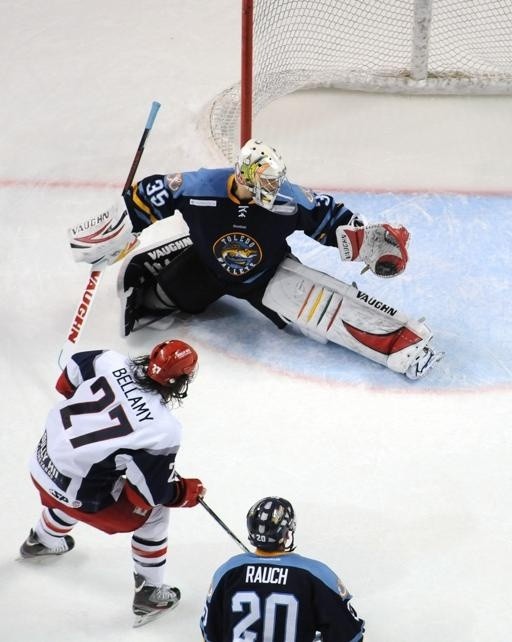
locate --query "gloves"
[166,477,205,509]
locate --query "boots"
[132,572,181,615]
[20,528,74,559]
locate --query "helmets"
[234,138,287,210]
[247,496,297,554]
[147,338,198,398]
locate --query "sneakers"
[406,345,433,381]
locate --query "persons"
[199,496,366,642]
[65,142,445,382]
[11,339,209,630]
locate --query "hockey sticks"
[173,472,297,554]
[58,101,160,372]
